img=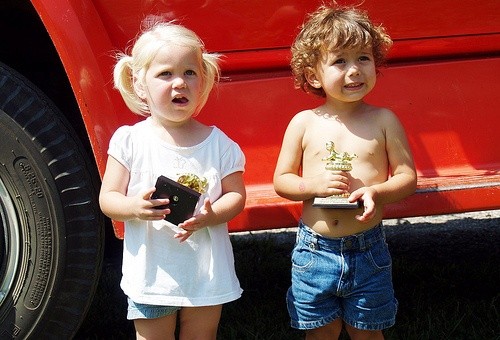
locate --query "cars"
[0,0,500,340]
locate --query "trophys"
[151,175,207,227]
[312,140,359,208]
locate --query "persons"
[99,24,245,340]
[271,8,418,340]
